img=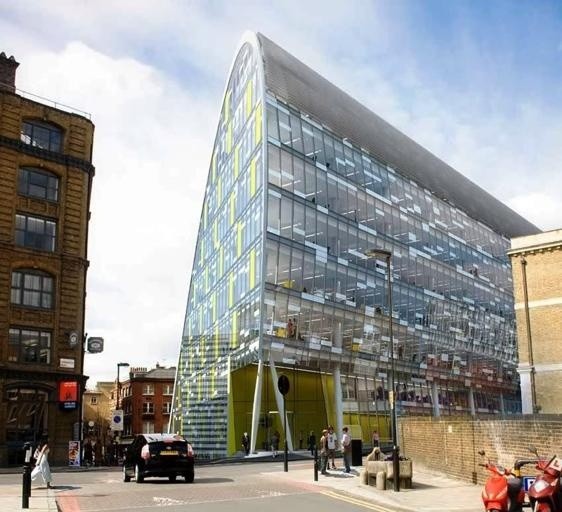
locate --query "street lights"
[116,362,129,409]
[365,248,400,490]
[89,420,95,436]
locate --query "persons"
[274,430,280,450]
[31,438,55,490]
[84,440,95,469]
[93,439,106,467]
[319,429,330,475]
[341,427,351,473]
[107,439,119,466]
[384,446,402,461]
[327,424,337,469]
[307,429,316,451]
[241,432,251,455]
[373,430,379,448]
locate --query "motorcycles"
[478,443,562,512]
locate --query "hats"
[42,432,48,436]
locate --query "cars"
[122,433,195,483]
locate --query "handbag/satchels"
[34,448,41,459]
